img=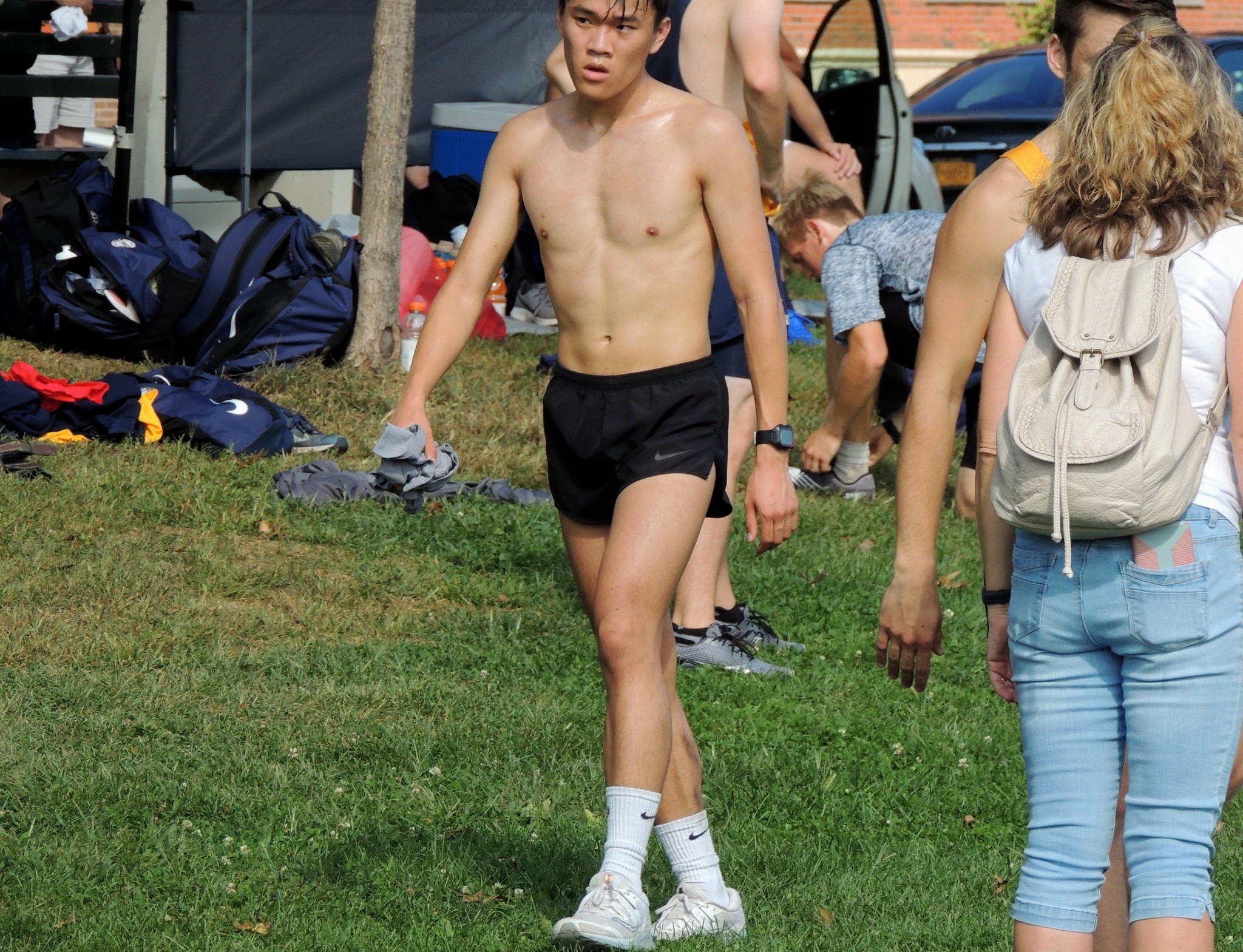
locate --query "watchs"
[754,424,794,449]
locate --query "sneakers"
[509,282,559,326]
[789,456,875,497]
[673,623,796,676]
[285,411,349,457]
[714,603,806,653]
[651,882,748,944]
[552,871,656,950]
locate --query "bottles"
[427,239,507,317]
[400,302,427,375]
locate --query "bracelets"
[982,587,1011,604]
[883,415,901,444]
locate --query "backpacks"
[34,198,217,363]
[0,152,118,340]
[990,214,1242,579]
[191,190,367,370]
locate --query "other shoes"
[787,309,825,346]
[311,229,344,263]
[0,440,57,482]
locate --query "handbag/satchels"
[135,365,294,458]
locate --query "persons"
[407,28,864,340]
[877,0,1243,952]
[27,0,100,149]
[547,0,786,680]
[770,176,992,521]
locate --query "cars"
[801,0,916,216]
[901,29,1243,213]
